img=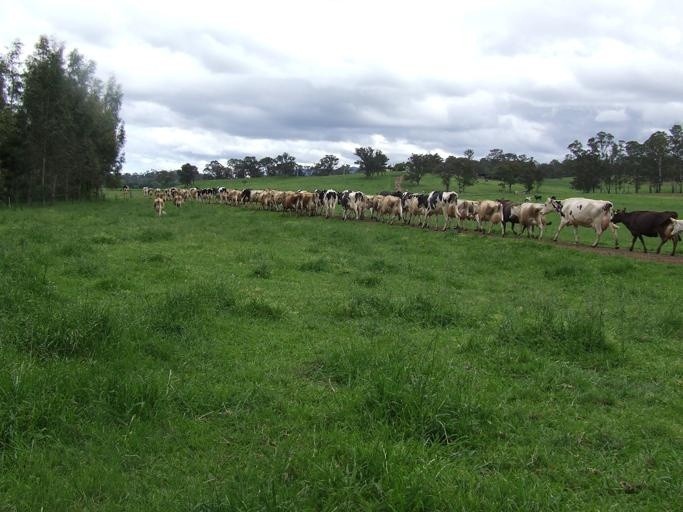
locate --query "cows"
[336,189,552,241]
[665,218,683,239]
[610,207,681,256]
[540,196,620,249]
[122,185,129,193]
[142,186,337,219]
